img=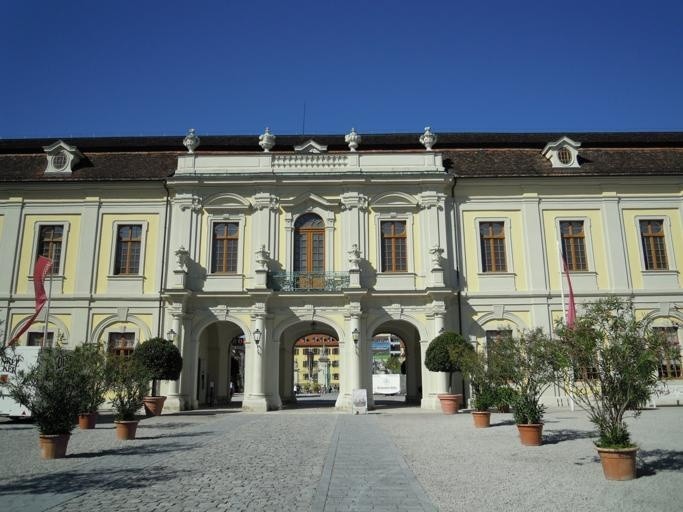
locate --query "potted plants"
[1,335,183,458]
